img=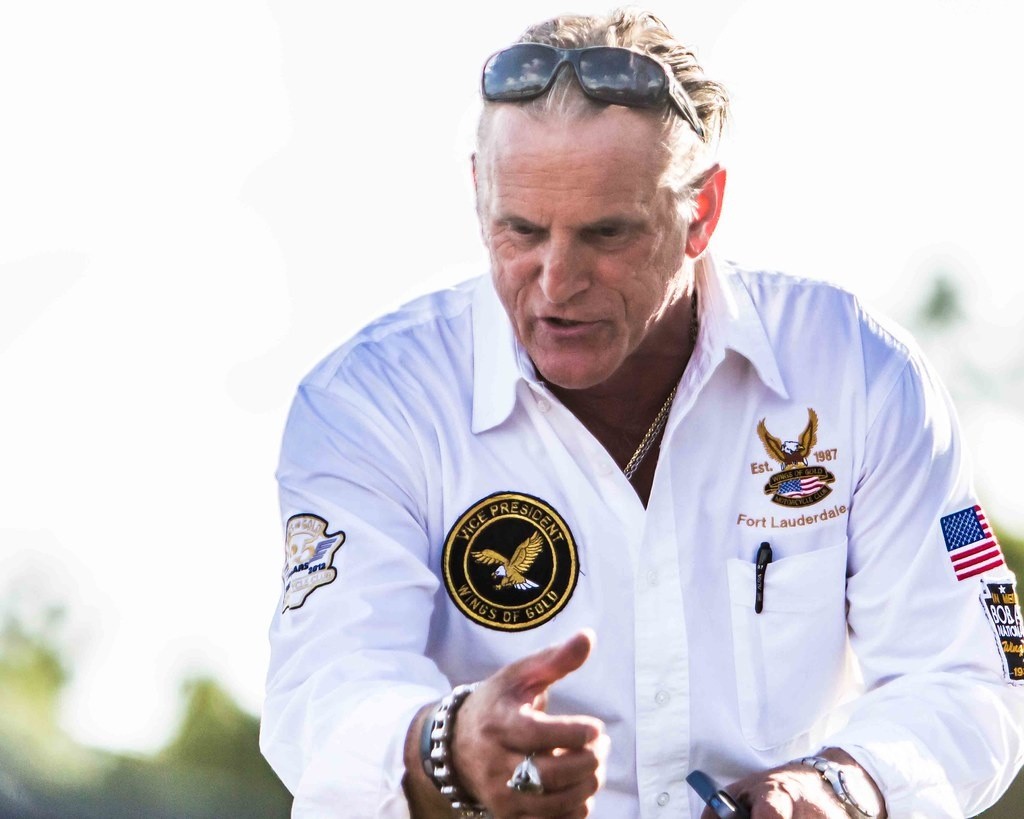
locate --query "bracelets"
[419,703,442,791]
[431,681,490,819]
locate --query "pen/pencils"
[754,541,772,613]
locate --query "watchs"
[789,757,881,819]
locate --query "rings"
[504,754,544,797]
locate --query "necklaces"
[623,290,698,479]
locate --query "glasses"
[481,41,703,136]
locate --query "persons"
[257,6,1024,819]
